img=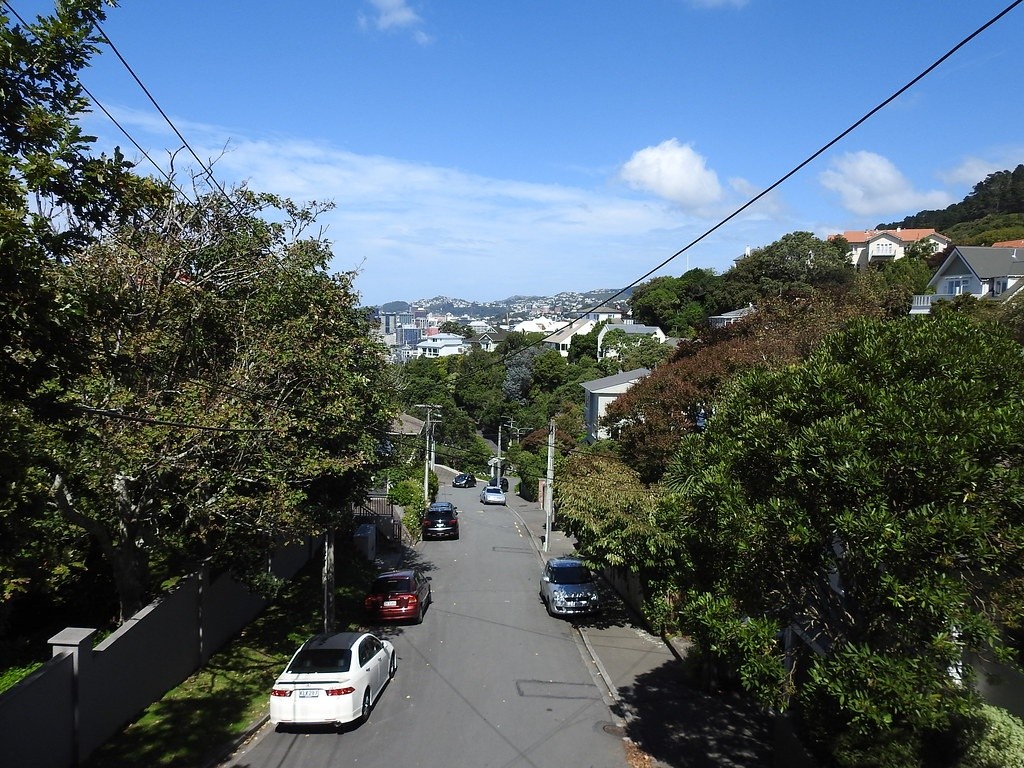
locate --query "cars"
[480,486,506,506]
[422,502,459,540]
[268,631,397,727]
[487,478,509,492]
[365,569,432,625]
[452,473,476,488]
[540,558,598,617]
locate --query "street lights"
[502,415,532,444]
